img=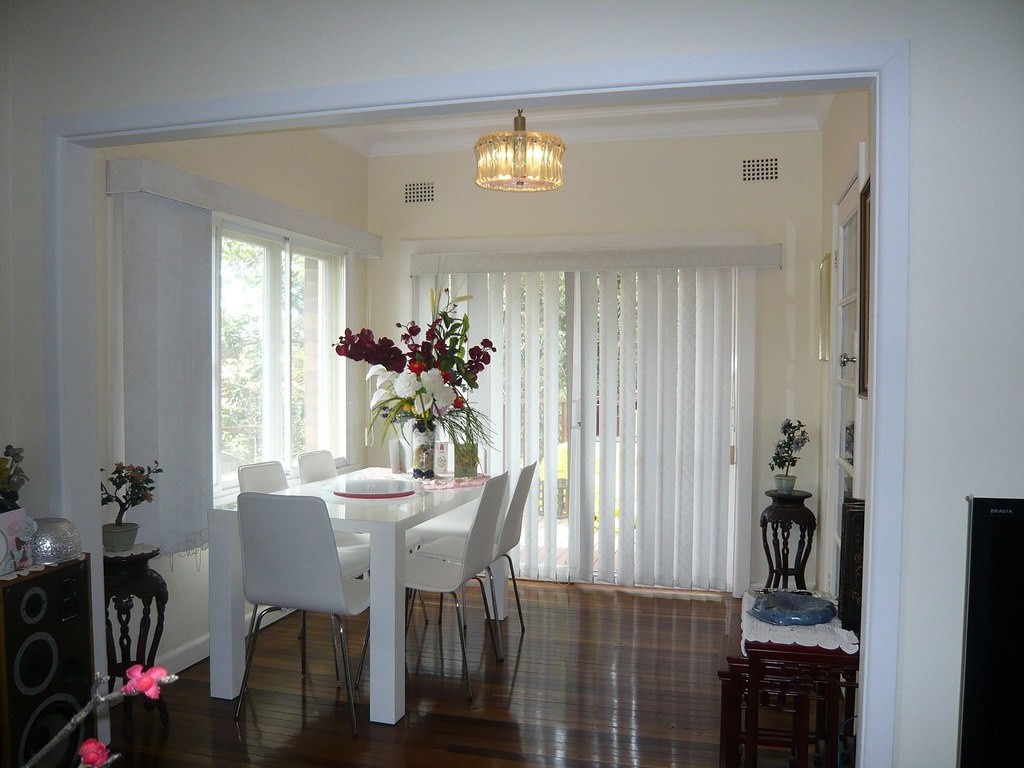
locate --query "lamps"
[473,109,565,193]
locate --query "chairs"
[233,491,371,740]
[298,449,373,546]
[353,471,509,701]
[410,459,538,664]
[238,460,371,680]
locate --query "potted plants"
[0,444,38,576]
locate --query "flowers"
[332,249,504,478]
[99,459,164,528]
[845,420,855,457]
[768,416,810,477]
[21,663,178,767]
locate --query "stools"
[716,615,841,768]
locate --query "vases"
[410,419,436,481]
[846,457,854,465]
[103,521,139,554]
[775,474,796,491]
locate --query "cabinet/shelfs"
[0,551,101,768]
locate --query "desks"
[208,465,489,724]
[741,589,862,768]
[103,543,169,744]
[760,489,817,593]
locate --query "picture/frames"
[818,253,830,361]
[856,174,871,400]
